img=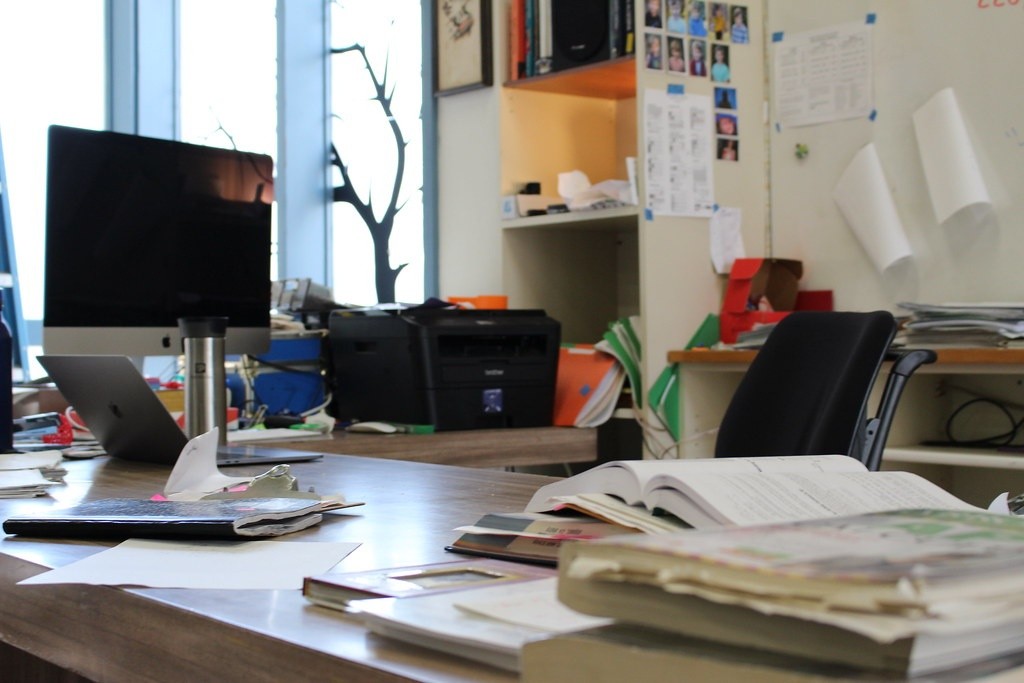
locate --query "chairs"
[713,309,938,474]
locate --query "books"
[550,340,627,429]
[510,0,637,80]
[301,454,1024,683]
[1,496,328,540]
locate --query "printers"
[330,308,562,434]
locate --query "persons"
[644,0,749,162]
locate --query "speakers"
[548,0,625,75]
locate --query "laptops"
[35,356,323,467]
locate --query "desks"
[0,422,1024,683]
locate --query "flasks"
[180,317,229,446]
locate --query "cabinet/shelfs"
[665,345,1024,508]
[492,0,774,482]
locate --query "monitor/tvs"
[42,124,273,375]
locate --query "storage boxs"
[225,329,331,430]
[328,307,562,432]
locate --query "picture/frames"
[430,0,494,98]
